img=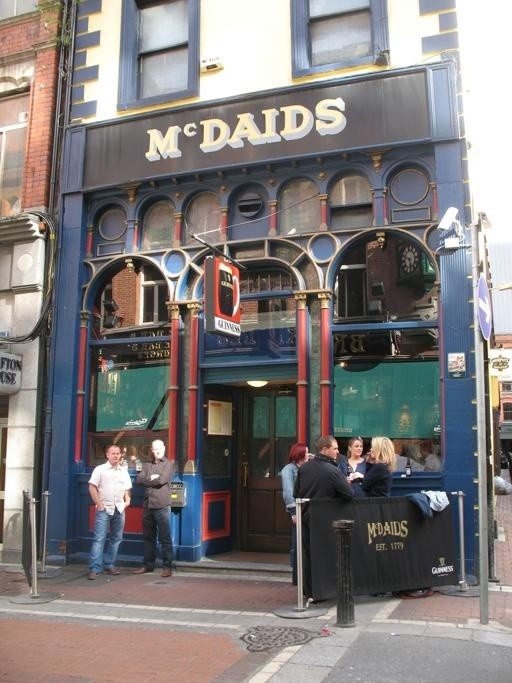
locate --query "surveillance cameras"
[436,207,463,237]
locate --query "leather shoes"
[132,566,151,574]
[160,569,172,577]
[89,571,100,581]
[104,568,121,575]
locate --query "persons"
[391,438,425,472]
[131,438,175,577]
[281,435,397,604]
[87,443,134,580]
[418,441,440,472]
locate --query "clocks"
[395,240,423,284]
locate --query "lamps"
[102,298,120,312]
[247,380,269,388]
[372,47,391,68]
[102,315,120,328]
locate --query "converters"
[444,237,460,250]
